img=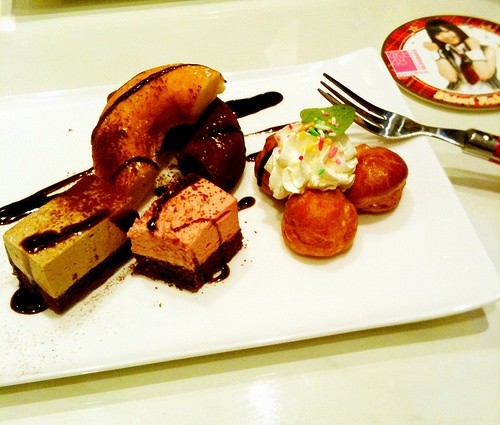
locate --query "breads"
[343,144,408,213]
[280,186,359,258]
[254,133,277,196]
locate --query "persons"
[421,18,500,95]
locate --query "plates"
[382,15,500,111]
[0,46,500,390]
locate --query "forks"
[316,73,500,166]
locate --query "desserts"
[3,157,181,316]
[127,175,243,292]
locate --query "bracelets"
[464,48,487,62]
[430,50,446,61]
